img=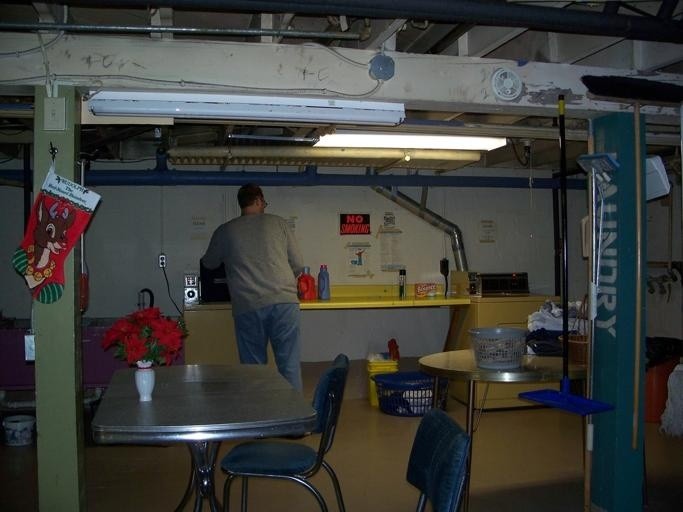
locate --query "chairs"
[405,375,474,512]
[285,353,350,453]
[219,367,346,512]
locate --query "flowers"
[100,305,186,368]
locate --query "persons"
[201,184,306,437]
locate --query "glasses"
[260,198,268,210]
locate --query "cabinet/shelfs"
[444,293,564,412]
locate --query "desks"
[91,363,318,512]
[417,349,587,512]
[180,297,472,384]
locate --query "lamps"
[88,90,404,128]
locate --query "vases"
[133,357,156,402]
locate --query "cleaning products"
[318,265,330,300]
[297,266,317,299]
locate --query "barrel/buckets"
[366,359,400,408]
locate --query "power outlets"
[159,255,165,268]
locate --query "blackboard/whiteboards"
[79,168,590,317]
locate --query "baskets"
[370,371,449,418]
[464,326,528,370]
[557,293,589,365]
